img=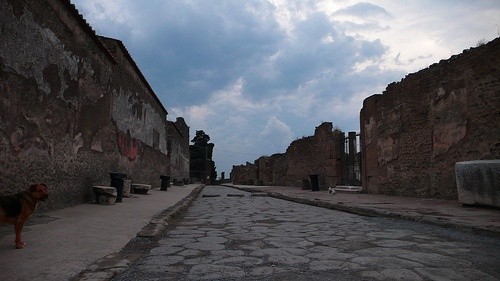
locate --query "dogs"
[0,182,49,249]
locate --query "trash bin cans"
[159,174,170,190]
[309,173,320,191]
[108,171,128,203]
